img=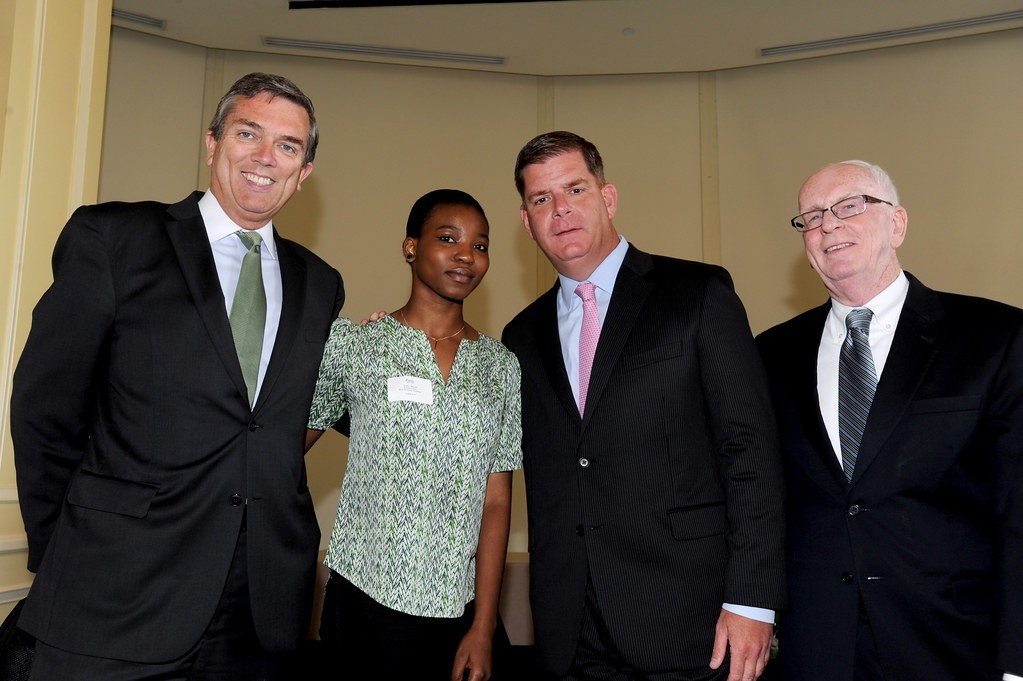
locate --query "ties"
[838,307,879,483]
[228,230,267,410]
[574,281,602,421]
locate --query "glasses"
[791,194,893,232]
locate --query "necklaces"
[400,305,466,350]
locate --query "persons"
[753,159,1023,680]
[301,186,525,680]
[357,131,789,680]
[8,70,351,681]
